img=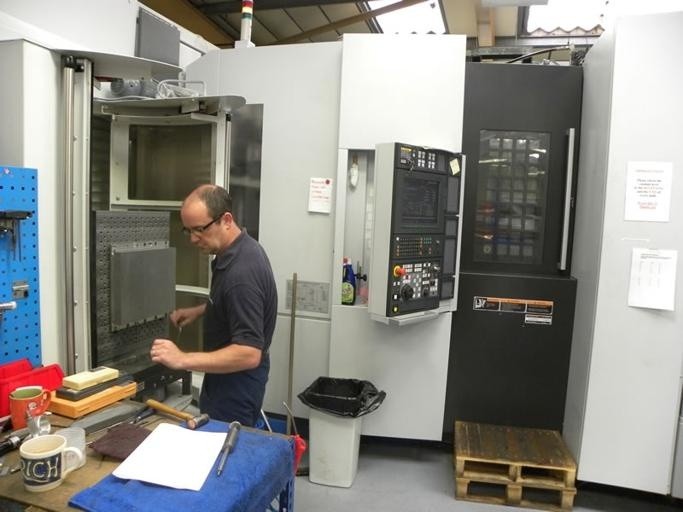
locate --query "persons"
[148,182,278,427]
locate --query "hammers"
[145,398,210,430]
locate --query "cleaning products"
[342,257,356,305]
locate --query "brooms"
[285,272,309,477]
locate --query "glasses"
[179,210,225,237]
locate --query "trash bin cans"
[297,376,386,488]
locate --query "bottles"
[342,256,356,305]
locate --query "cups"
[19,432,83,493]
[54,428,87,471]
[9,388,50,431]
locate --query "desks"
[0,412,296,512]
